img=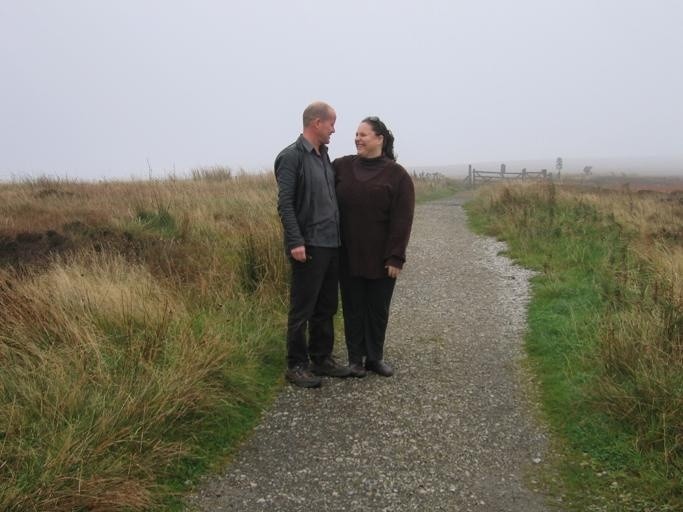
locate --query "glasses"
[360,116,382,127]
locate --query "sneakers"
[307,357,351,377]
[284,365,321,388]
[347,363,367,378]
[365,358,392,379]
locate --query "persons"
[271,102,353,391]
[331,116,414,378]
